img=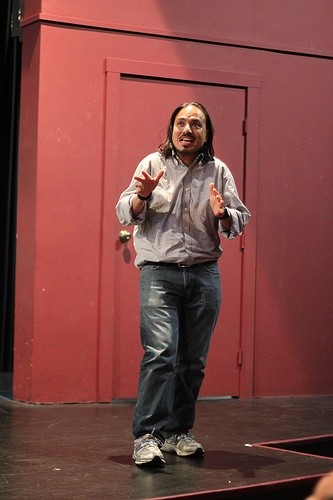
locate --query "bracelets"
[219,208,229,220]
[138,192,152,200]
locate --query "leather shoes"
[146,260,217,270]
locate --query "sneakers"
[132,430,206,468]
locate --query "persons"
[114,101,251,468]
